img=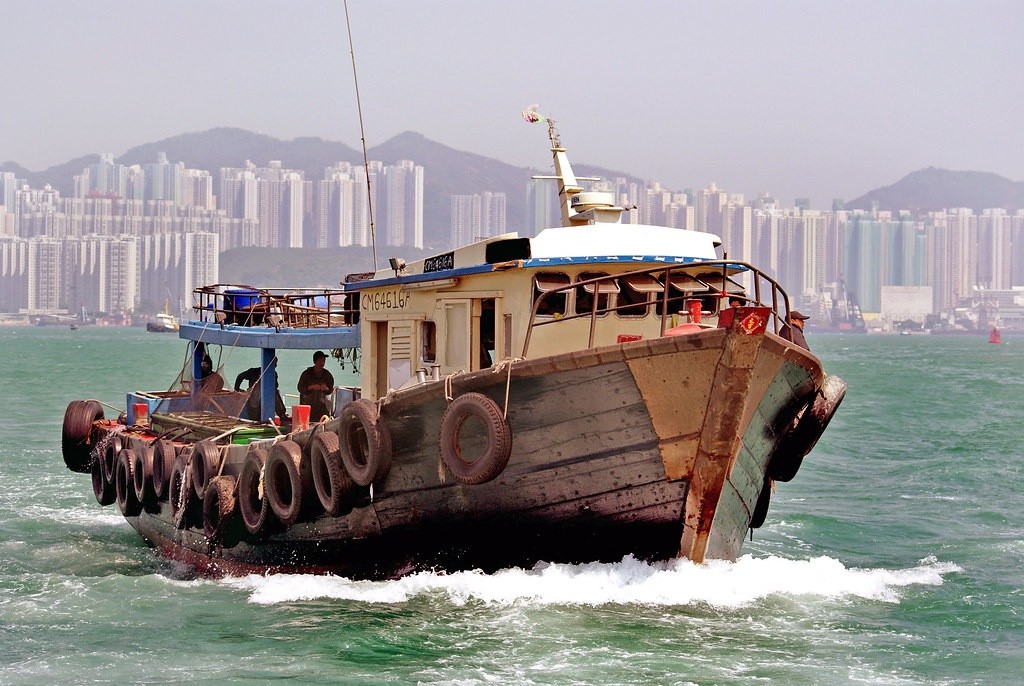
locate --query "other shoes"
[280,416,292,422]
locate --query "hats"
[785,311,811,322]
[313,351,328,362]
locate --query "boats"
[146,313,180,332]
[62,104,847,579]
[39,314,78,325]
[70,324,80,330]
[900,331,911,335]
[928,284,1024,333]
[799,272,868,335]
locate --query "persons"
[717,292,746,327]
[779,311,811,352]
[234,356,292,422]
[296,350,335,422]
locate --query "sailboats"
[989,327,1001,343]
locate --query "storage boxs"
[207,284,263,327]
[127,389,248,426]
